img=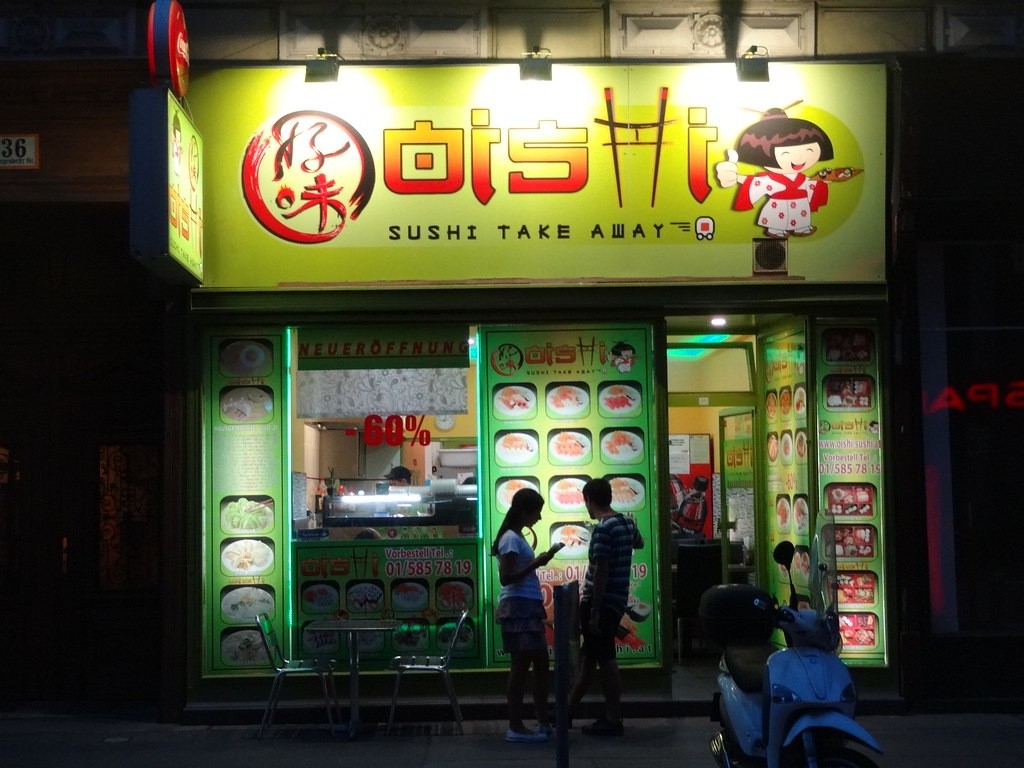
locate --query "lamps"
[304,48,347,82]
[519,46,552,81]
[736,45,772,82]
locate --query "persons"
[457,476,476,537]
[384,466,411,485]
[567,480,645,737]
[491,489,552,742]
[357,528,380,540]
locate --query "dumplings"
[224,497,268,528]
[222,540,269,570]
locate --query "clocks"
[434,415,455,431]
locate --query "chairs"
[669,538,742,666]
[383,608,469,736]
[256,612,344,739]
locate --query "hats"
[383,466,411,481]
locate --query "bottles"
[670,473,686,522]
[677,475,708,534]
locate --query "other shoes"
[505,729,548,742]
[581,716,624,738]
[549,710,573,728]
[539,723,554,736]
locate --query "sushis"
[307,632,337,648]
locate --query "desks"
[304,618,405,736]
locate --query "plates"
[221,500,274,663]
[302,581,471,653]
[494,385,644,555]
[765,387,810,580]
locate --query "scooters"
[694,541,885,768]
